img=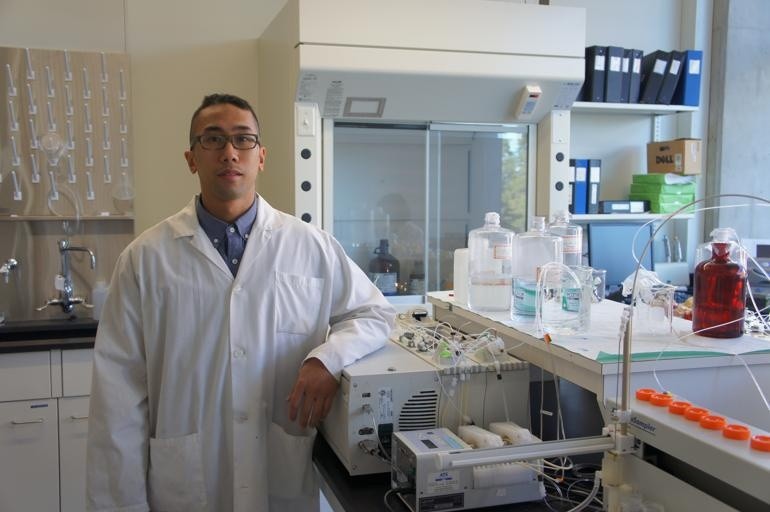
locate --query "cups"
[634,286,677,336]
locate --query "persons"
[84,92,396,512]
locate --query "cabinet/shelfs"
[48,342,96,510]
[568,100,700,289]
[2,352,62,512]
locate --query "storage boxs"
[646,136,702,176]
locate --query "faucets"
[34,238,96,312]
[1,258,18,284]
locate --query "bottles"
[405,257,427,295]
[689,240,750,337]
[467,213,618,334]
[91,280,109,321]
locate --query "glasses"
[190,134,260,150]
